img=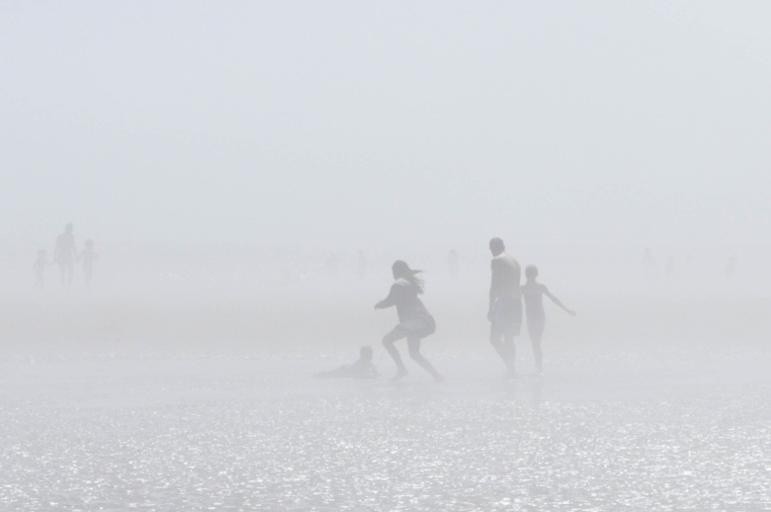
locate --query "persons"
[372,259,450,382]
[520,264,575,369]
[484,234,522,372]
